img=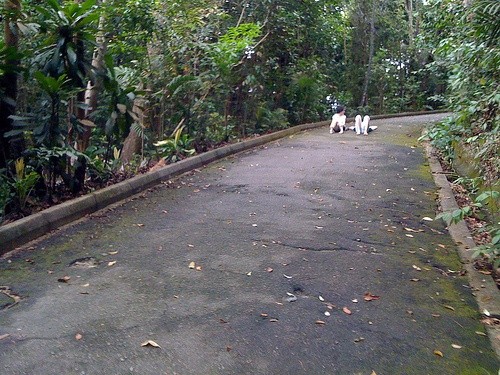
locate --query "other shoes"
[329,129,344,134]
[354,132,368,136]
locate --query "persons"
[330,106,348,134]
[349,114,378,135]
